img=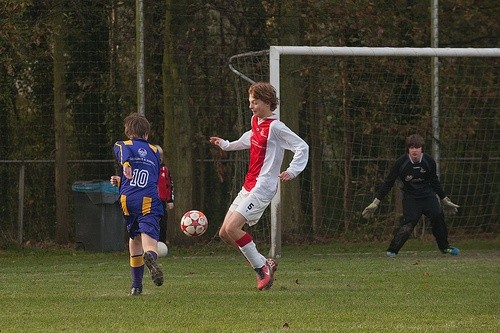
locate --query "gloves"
[441,197,460,216]
[362,198,381,220]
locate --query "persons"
[210,82,310,289]
[158,158,175,243]
[109,113,163,296]
[362,134,460,257]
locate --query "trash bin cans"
[72,179,127,253]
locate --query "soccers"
[180,209,208,239]
[157,241,168,258]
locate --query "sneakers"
[141,252,166,287]
[130,283,142,297]
[441,246,461,256]
[386,250,397,258]
[254,257,278,291]
